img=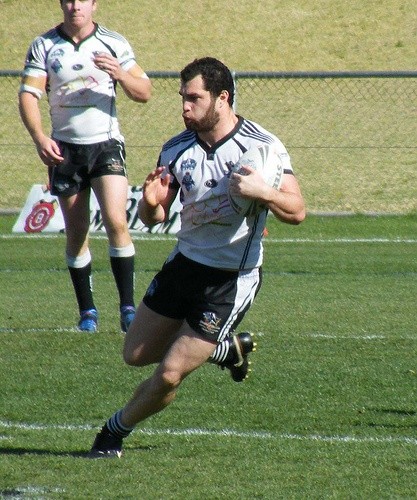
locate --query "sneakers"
[120,305,136,332]
[227,329,257,381]
[89,424,124,458]
[77,309,99,333]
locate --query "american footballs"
[226,143,286,218]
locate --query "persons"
[18,0,151,334]
[91,58,305,457]
